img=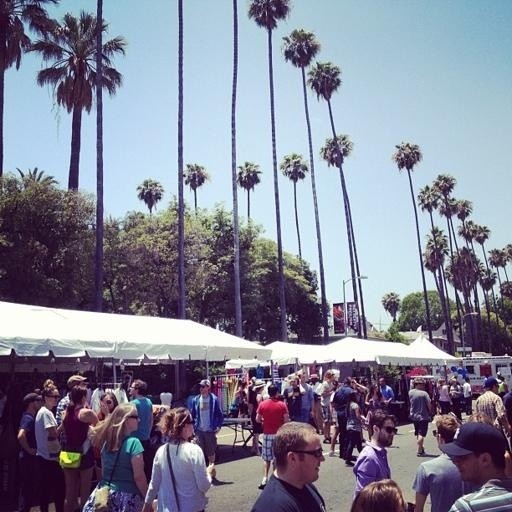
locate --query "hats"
[66,375,87,386]
[440,421,506,457]
[412,377,426,385]
[197,379,211,387]
[23,393,42,403]
[482,376,501,387]
[252,379,266,391]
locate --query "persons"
[14,368,512,512]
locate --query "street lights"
[342,273,370,339]
[461,311,483,359]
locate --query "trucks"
[424,353,512,400]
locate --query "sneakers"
[208,475,225,485]
[416,449,429,457]
[323,436,366,467]
[258,482,267,490]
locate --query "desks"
[221,417,252,454]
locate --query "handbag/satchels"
[58,451,81,469]
[82,480,119,512]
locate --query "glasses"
[290,447,322,458]
[105,400,113,405]
[381,426,399,434]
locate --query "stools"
[241,425,262,448]
